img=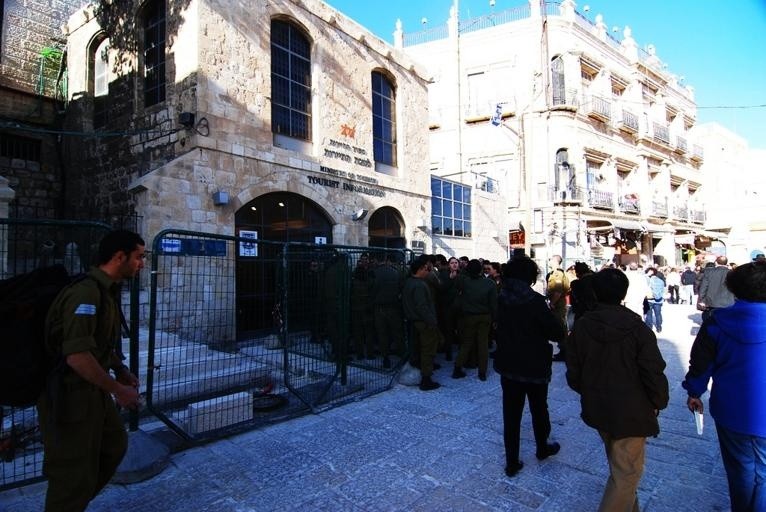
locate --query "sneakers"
[536,443,560,460]
[505,460,523,476]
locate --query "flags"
[486,101,507,126]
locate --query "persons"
[682,259,766,510]
[545,256,571,361]
[574,262,667,332]
[34,227,147,511]
[305,253,500,392]
[563,267,671,512]
[489,255,563,478]
[667,256,737,312]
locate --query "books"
[693,403,705,438]
